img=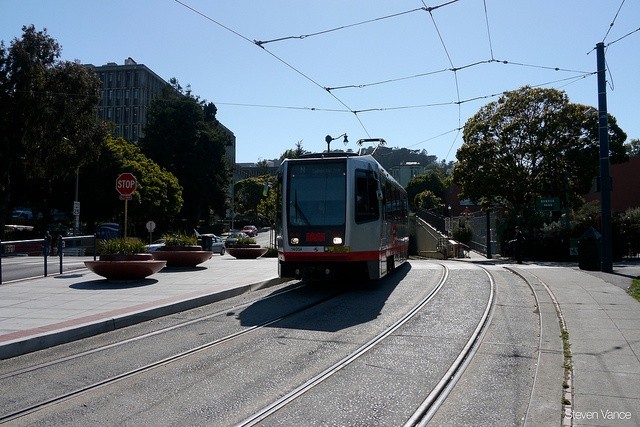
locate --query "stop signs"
[116,173,137,196]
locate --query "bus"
[276,152,409,295]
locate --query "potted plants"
[227,239,271,260]
[156,234,213,275]
[83,238,166,286]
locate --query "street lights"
[325,133,349,153]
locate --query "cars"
[241,225,258,237]
[225,233,250,248]
[197,233,225,256]
